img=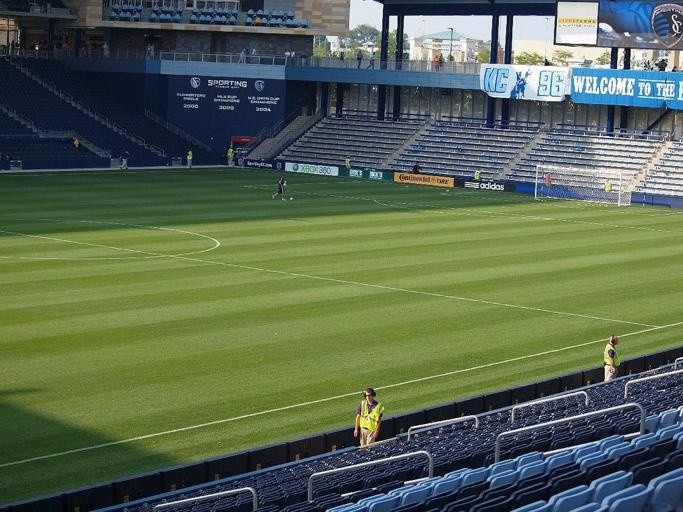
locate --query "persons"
[604,336,621,381]
[367,52,376,68]
[545,58,549,66]
[356,50,363,69]
[510,80,525,100]
[657,59,667,71]
[4,38,155,60]
[345,155,351,175]
[474,168,480,181]
[237,47,256,63]
[187,149,193,168]
[119,150,130,170]
[411,161,422,174]
[545,172,552,187]
[280,48,297,63]
[272,175,287,201]
[72,136,81,148]
[353,387,384,446]
[227,146,244,166]
[327,50,344,67]
[604,180,612,193]
[434,53,443,71]
[672,66,677,72]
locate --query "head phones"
[364,388,376,398]
[611,335,615,344]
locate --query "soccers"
[290,196,294,200]
